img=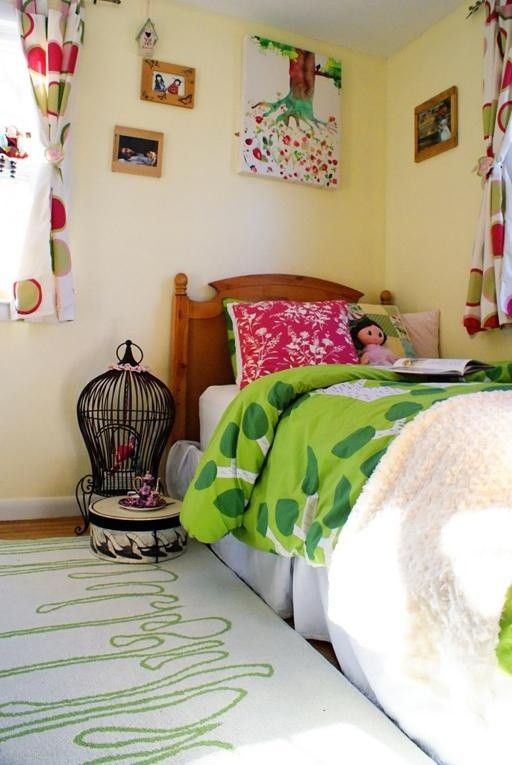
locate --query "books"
[370,357,496,376]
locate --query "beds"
[167,269,512,764]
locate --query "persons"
[352,319,397,369]
[154,74,181,94]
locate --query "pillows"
[220,296,441,390]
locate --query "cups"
[146,491,160,508]
[127,491,137,506]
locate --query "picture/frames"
[413,85,458,164]
[112,125,163,178]
[140,57,195,109]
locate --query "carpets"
[0,536,438,765]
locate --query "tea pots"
[133,469,161,505]
